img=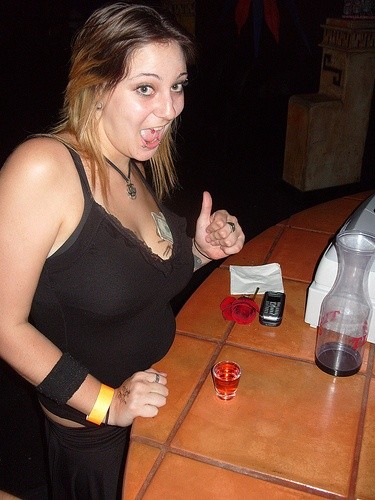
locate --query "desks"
[121,189,374,500]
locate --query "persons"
[0,0,244,500]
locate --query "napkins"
[229,262,285,294]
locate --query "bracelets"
[31,349,90,407]
[105,408,109,427]
[194,237,216,262]
[87,383,115,425]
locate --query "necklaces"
[103,153,137,201]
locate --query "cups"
[212,359,242,400]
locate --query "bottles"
[315,231,375,376]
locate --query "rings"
[155,373,160,383]
[226,222,236,232]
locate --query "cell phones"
[258,291,286,328]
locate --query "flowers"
[221,287,260,325]
